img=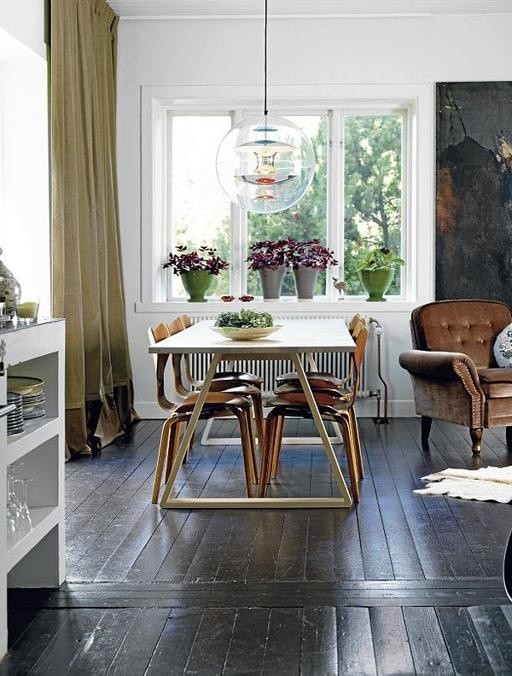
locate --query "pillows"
[493,322,512,367]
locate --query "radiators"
[186,315,389,424]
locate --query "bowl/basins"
[207,324,283,342]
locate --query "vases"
[179,269,213,302]
[257,265,287,299]
[290,264,319,299]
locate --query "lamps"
[216,1,316,216]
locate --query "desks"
[148,319,356,509]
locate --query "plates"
[7,375,47,438]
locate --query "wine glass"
[7,462,33,547]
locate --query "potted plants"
[353,243,408,302]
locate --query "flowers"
[162,243,230,275]
[244,235,339,271]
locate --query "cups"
[1,292,7,328]
[11,295,42,323]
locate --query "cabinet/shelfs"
[1,316,67,658]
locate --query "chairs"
[166,319,264,485]
[400,298,512,457]
[260,322,368,501]
[263,319,367,479]
[148,322,252,505]
[177,314,265,456]
[275,315,360,455]
